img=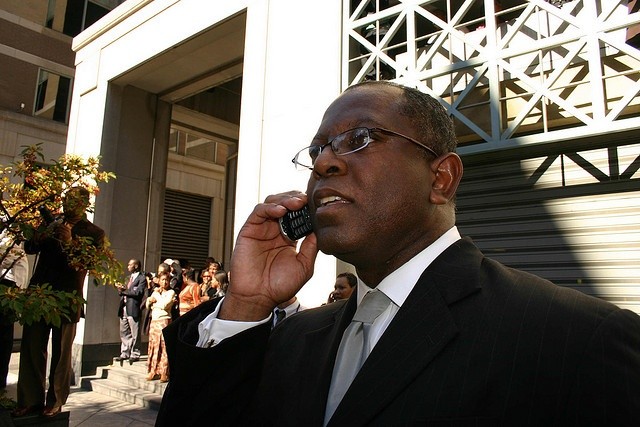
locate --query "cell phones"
[276,203,314,246]
[333,293,336,300]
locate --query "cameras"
[38,205,68,230]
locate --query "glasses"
[202,276,210,277]
[291,127,438,170]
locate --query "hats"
[164,259,175,266]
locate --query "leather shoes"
[129,357,139,362]
[11,404,44,417]
[116,357,129,361]
[42,406,61,418]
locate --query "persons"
[14,189,105,415]
[114,256,231,381]
[324,272,357,302]
[270,294,308,331]
[155,82,549,424]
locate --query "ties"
[323,291,392,427]
[275,310,286,324]
[123,277,133,303]
